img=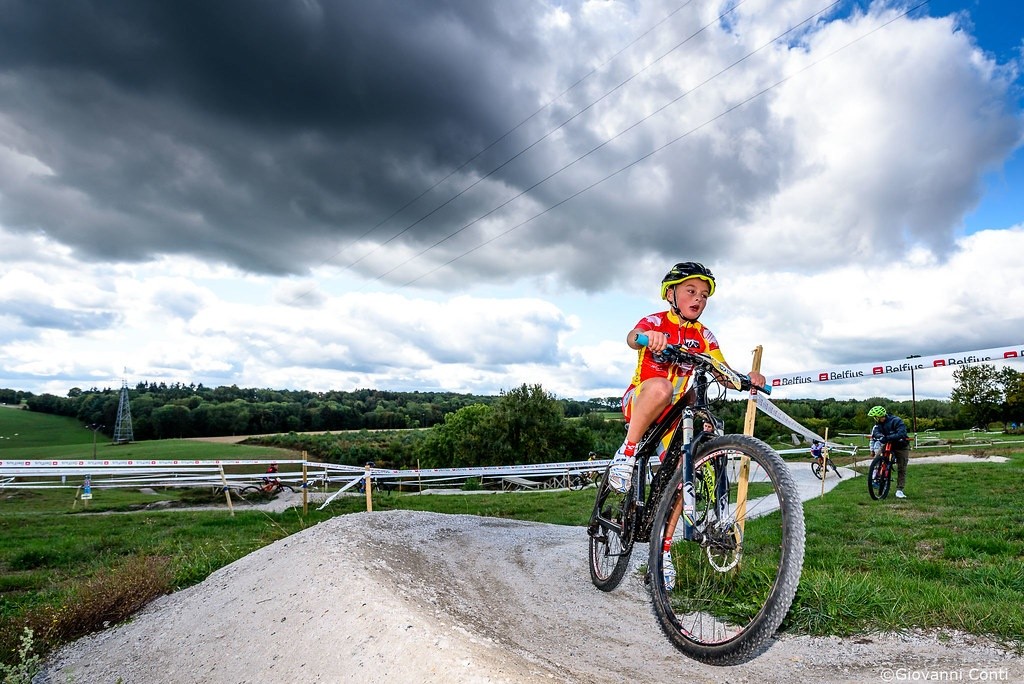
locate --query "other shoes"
[817,468,820,471]
[827,469,829,472]
[872,470,878,479]
[896,492,907,498]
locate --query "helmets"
[867,406,887,423]
[813,439,819,443]
[661,262,716,299]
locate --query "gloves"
[815,456,818,458]
[830,447,832,449]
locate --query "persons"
[703,420,729,517]
[869,420,887,489]
[866,406,910,498]
[810,439,833,473]
[258,463,281,498]
[585,452,597,483]
[359,461,376,494]
[608,261,767,593]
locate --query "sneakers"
[608,450,636,493]
[663,551,677,590]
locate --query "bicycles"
[343,477,390,496]
[811,448,842,480]
[587,333,807,668]
[241,477,293,498]
[865,434,915,501]
[567,471,604,491]
